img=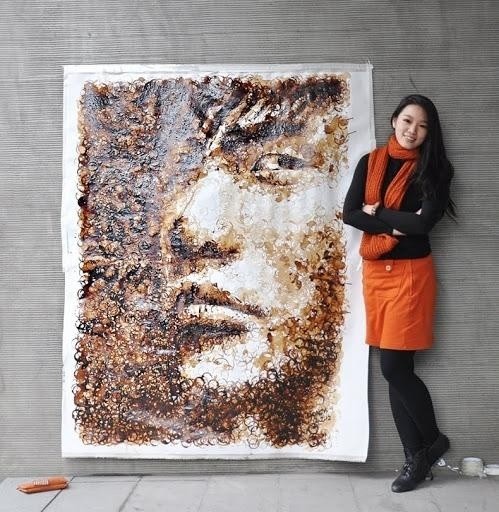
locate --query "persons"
[158,79,342,443]
[342,95,458,492]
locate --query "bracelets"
[370,206,376,218]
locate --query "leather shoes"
[392,433,449,492]
